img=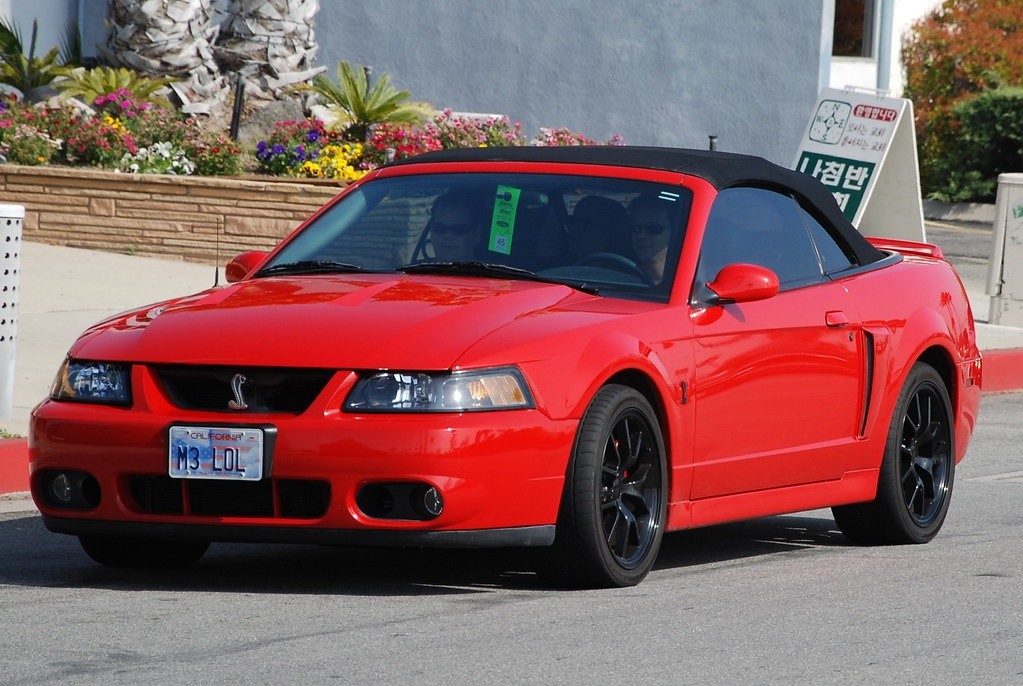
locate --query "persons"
[431,192,484,260]
[621,196,680,295]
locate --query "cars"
[27,140,983,588]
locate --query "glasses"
[430,219,475,236]
[629,224,665,236]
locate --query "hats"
[565,195,628,232]
